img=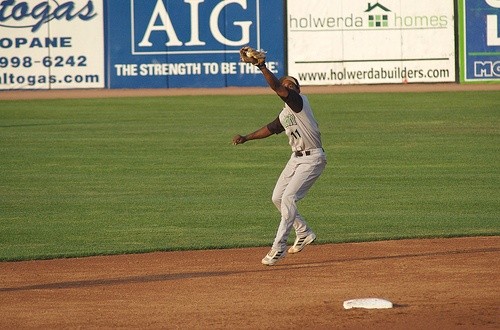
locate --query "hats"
[279,76,299,87]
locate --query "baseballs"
[246,52,252,58]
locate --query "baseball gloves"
[239,47,266,66]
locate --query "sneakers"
[288,234,316,253]
[261,246,286,266]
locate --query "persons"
[233,46,326,266]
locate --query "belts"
[295,149,324,156]
